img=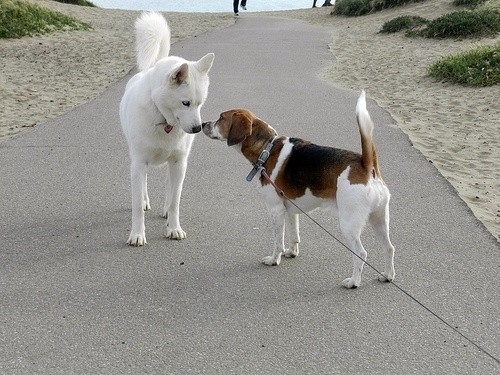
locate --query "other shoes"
[240,4,247,10]
[235,12,239,16]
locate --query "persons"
[233,0,248,17]
[312,0,333,8]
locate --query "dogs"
[119,10,215,247]
[202,88,395,290]
